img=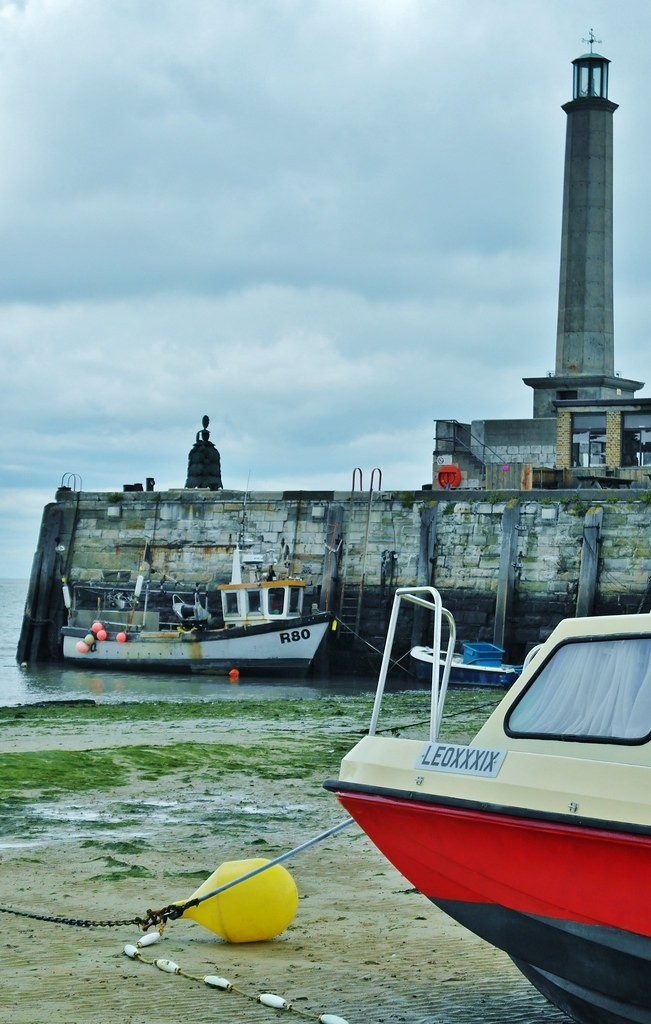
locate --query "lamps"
[312,507,325,518]
[541,509,557,520]
[108,507,121,517]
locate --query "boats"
[409,640,523,689]
[60,531,335,679]
[320,583,651,1024]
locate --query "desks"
[571,475,638,490]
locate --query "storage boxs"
[462,642,505,668]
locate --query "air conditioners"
[437,455,452,465]
